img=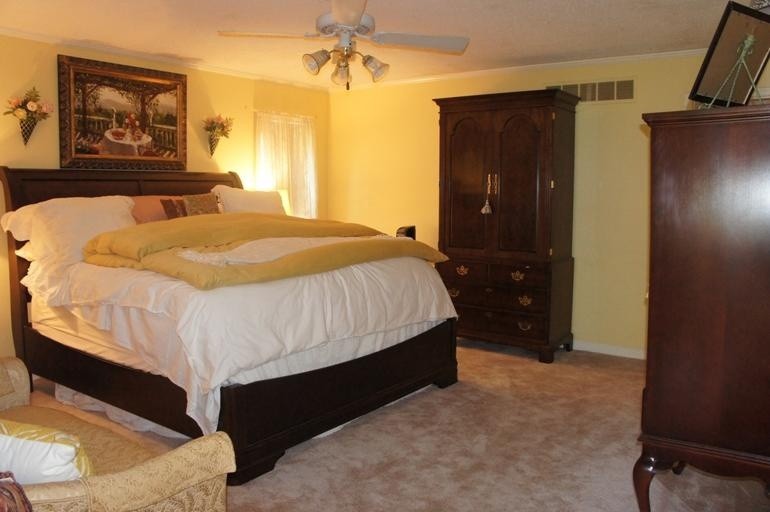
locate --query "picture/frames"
[57,53,188,170]
[688,0,770,110]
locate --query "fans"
[216,0,471,56]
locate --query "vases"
[208,133,220,157]
[18,118,36,145]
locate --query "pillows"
[1,184,286,274]
[0,419,96,484]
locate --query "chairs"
[0,357,239,512]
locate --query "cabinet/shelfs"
[431,255,574,364]
[631,103,770,512]
[434,86,584,254]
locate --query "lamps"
[302,47,390,92]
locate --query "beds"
[0,164,458,486]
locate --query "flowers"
[203,114,235,138]
[3,85,55,122]
